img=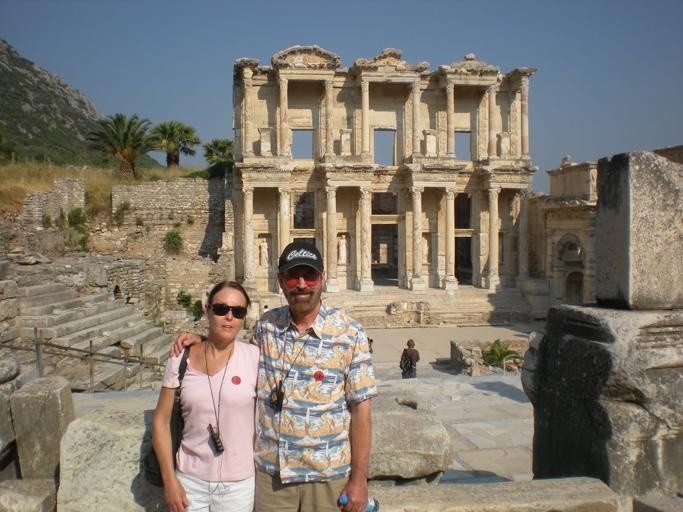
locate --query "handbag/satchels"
[400,349,412,371]
[143,399,184,488]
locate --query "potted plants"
[159,227,183,257]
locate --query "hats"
[278,241,323,274]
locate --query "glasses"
[283,274,324,288]
[205,303,247,319]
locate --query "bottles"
[340,497,379,512]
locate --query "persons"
[144,281,259,512]
[368,338,374,353]
[168,241,379,512]
[400,339,420,379]
[264,305,269,312]
